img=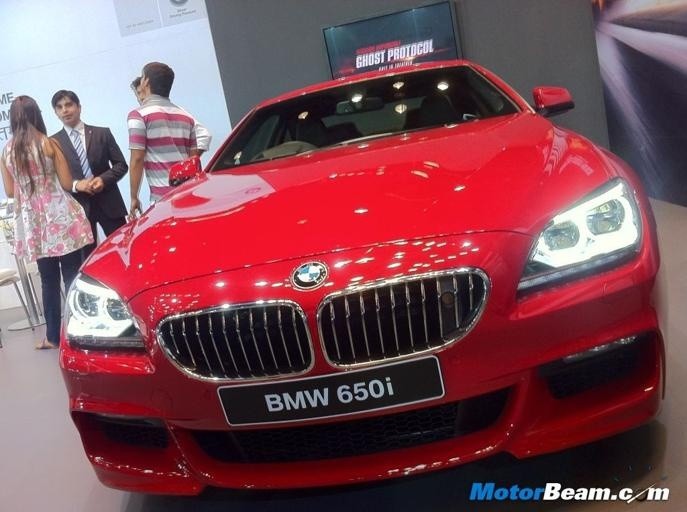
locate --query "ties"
[72,130,93,179]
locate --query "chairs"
[298,118,328,148]
[420,94,458,125]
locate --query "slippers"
[35,337,59,349]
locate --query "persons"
[48,89,128,259]
[128,61,203,220]
[0,94,95,351]
[131,76,213,157]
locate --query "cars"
[54,55,672,503]
